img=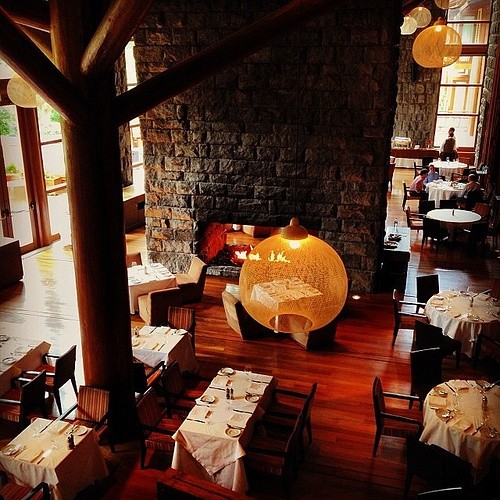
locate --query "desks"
[420,379,500,468]
[394,158,423,169]
[425,291,500,358]
[0,417,110,500]
[428,160,467,180]
[425,208,482,251]
[425,181,470,208]
[127,263,176,314]
[127,324,200,381]
[171,369,274,493]
[0,327,50,398]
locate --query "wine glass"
[445,285,494,321]
[30,417,44,438]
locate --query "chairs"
[371,274,500,500]
[0,251,350,500]
[402,152,497,256]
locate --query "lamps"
[7,74,46,108]
[238,0,348,334]
[400,0,467,68]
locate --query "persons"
[457,174,481,204]
[439,128,457,162]
[409,169,429,210]
[424,165,438,183]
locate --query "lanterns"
[239,234,348,333]
[7,74,45,109]
[400,7,432,35]
[412,25,462,68]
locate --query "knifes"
[187,418,205,423]
[233,409,253,414]
[252,380,269,384]
[13,445,26,458]
[39,420,53,432]
[209,386,225,391]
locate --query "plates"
[200,394,216,403]
[224,427,241,437]
[2,444,20,456]
[2,357,16,365]
[71,425,88,436]
[244,393,260,403]
[384,241,399,247]
[388,234,401,241]
[173,329,188,334]
[220,367,234,374]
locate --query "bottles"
[393,217,399,233]
[226,388,235,400]
[144,266,148,275]
[134,326,140,337]
